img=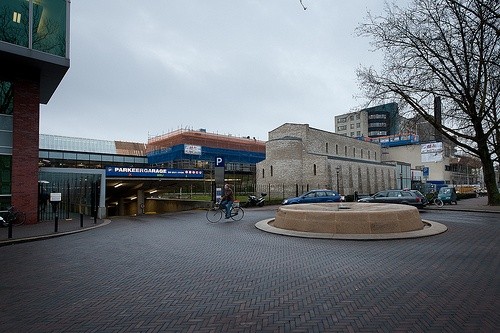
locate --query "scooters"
[244,191,267,208]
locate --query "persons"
[318,187,324,196]
[453,187,457,205]
[325,185,330,190]
[221,184,234,219]
[474,187,487,197]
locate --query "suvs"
[281,189,346,205]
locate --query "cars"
[437,186,457,207]
[358,188,430,209]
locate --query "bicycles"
[429,193,444,208]
[0,203,26,226]
[206,198,244,223]
